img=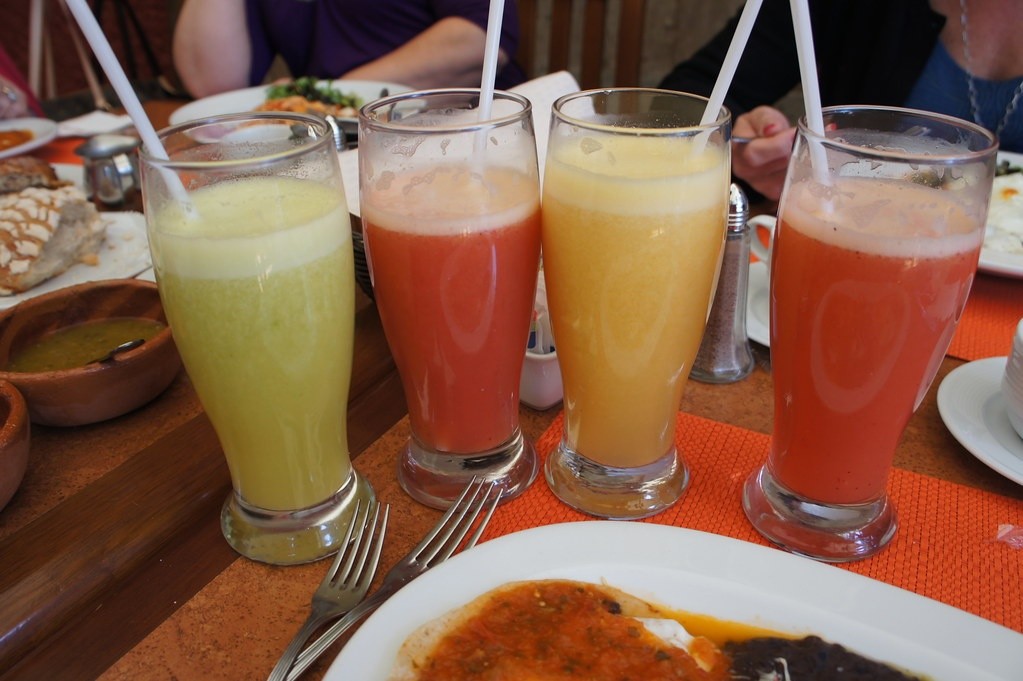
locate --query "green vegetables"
[262,78,362,109]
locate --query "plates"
[1,117,59,156]
[743,257,770,345]
[840,149,1023,276]
[49,163,93,200]
[0,212,154,308]
[937,356,1023,486]
[168,80,426,143]
[59,109,133,137]
[323,520,1023,681]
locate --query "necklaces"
[959,0,1023,136]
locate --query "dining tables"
[0,106,1023,681]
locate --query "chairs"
[517,0,648,114]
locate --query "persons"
[657,0,1023,201]
[172,0,520,111]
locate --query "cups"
[540,87,729,520]
[1003,318,1023,440]
[73,135,141,206]
[743,105,998,561]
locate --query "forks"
[267,499,389,681]
[286,475,503,681]
[731,124,931,142]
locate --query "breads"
[0,156,107,297]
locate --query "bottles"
[690,182,754,384]
[137,112,374,564]
[357,89,540,510]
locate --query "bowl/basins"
[0,380,30,510]
[0,280,181,423]
[519,351,562,410]
